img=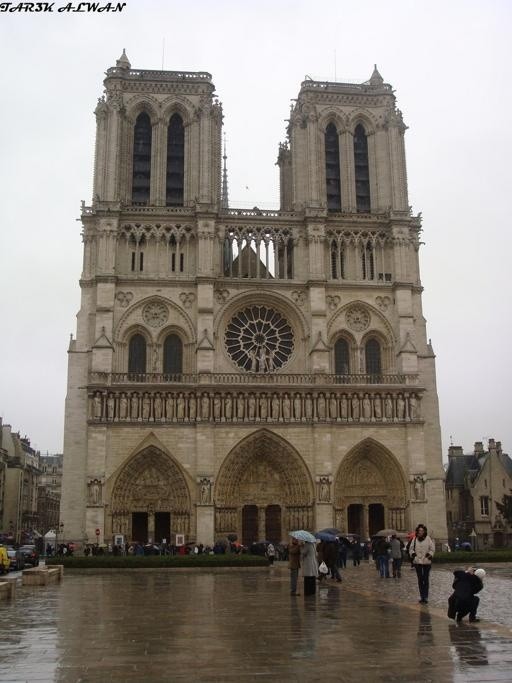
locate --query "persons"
[409,524,435,604]
[448,567,486,626]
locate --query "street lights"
[49,520,66,558]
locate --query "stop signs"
[95,528,100,535]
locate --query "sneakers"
[457,617,480,623]
[419,598,429,605]
[340,563,361,569]
[290,572,343,597]
[376,566,414,579]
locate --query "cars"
[137,542,177,555]
[250,540,271,555]
[0,542,39,575]
